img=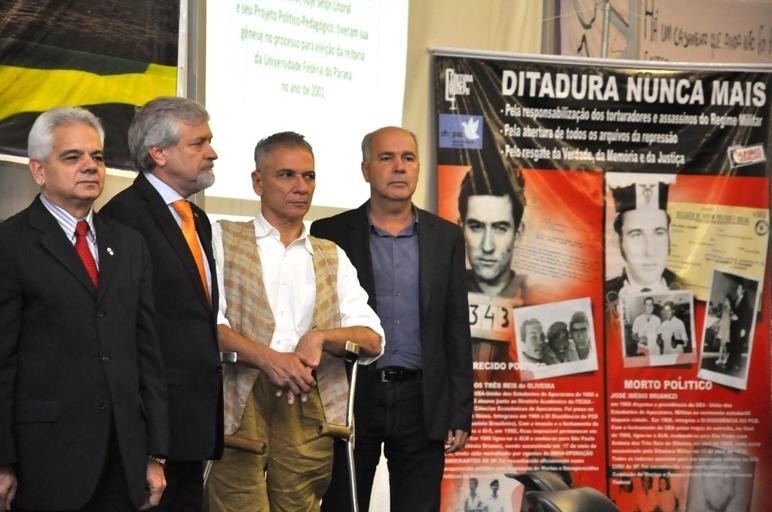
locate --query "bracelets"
[148,456,166,465]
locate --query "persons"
[710,282,752,364]
[458,164,550,380]
[464,477,502,512]
[98,97,218,512]
[606,182,688,325]
[618,478,676,512]
[310,126,473,512]
[211,132,386,512]
[633,297,688,354]
[0,105,169,511]
[521,311,590,364]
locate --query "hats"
[605,172,677,213]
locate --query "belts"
[374,366,423,383]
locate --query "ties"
[74,221,99,289]
[173,197,210,311]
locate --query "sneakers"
[723,352,730,365]
[715,359,723,365]
